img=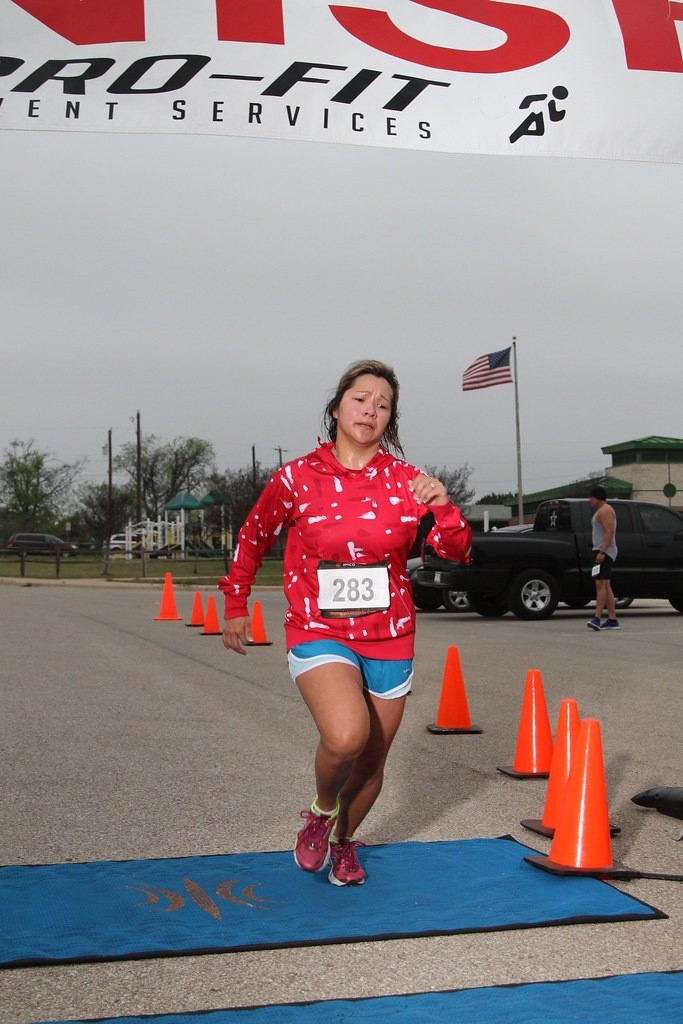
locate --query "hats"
[590,485,607,502]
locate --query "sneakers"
[324,834,366,887]
[292,791,341,874]
[586,617,601,632]
[598,619,620,630]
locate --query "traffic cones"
[425,645,485,735]
[185,591,204,627]
[495,669,554,779]
[243,599,273,647]
[153,572,184,619]
[520,700,621,838]
[523,718,641,877]
[200,595,224,634]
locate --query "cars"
[405,524,538,612]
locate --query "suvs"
[416,498,683,621]
[8,533,79,558]
[101,534,141,551]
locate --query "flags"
[461,344,514,393]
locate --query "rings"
[429,482,436,490]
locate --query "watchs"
[599,551,605,555]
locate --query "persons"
[586,486,622,631]
[216,359,474,887]
